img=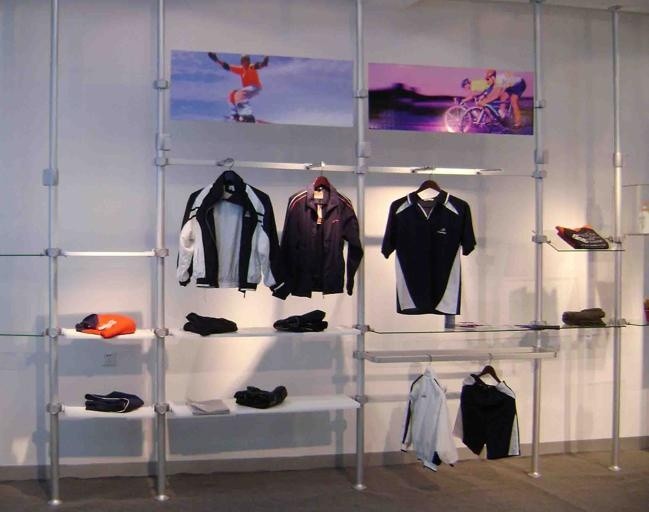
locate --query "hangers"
[416,168,441,193]
[314,166,330,190]
[223,158,235,182]
[476,353,501,384]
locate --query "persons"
[477,69,527,129]
[460,78,512,120]
[207,52,270,123]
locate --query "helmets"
[485,71,496,79]
[462,79,469,88]
[241,55,250,63]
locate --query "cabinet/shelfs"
[42,0,649,506]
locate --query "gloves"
[209,53,218,62]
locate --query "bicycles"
[444,96,533,135]
